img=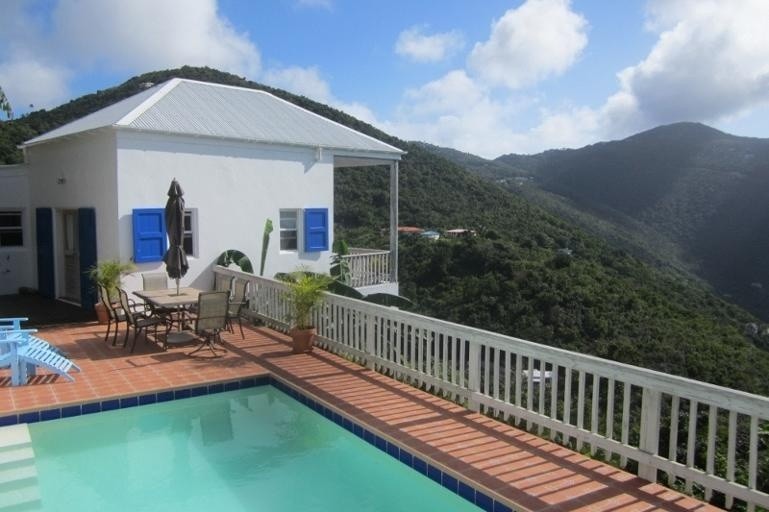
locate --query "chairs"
[223,276,235,291]
[188,290,231,358]
[228,277,249,339]
[0,317,65,356]
[0,329,81,386]
[96,282,153,346]
[141,272,168,290]
[114,286,172,353]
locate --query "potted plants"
[83,259,136,324]
[277,260,342,353]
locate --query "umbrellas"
[161,176,190,332]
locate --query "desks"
[132,287,198,351]
